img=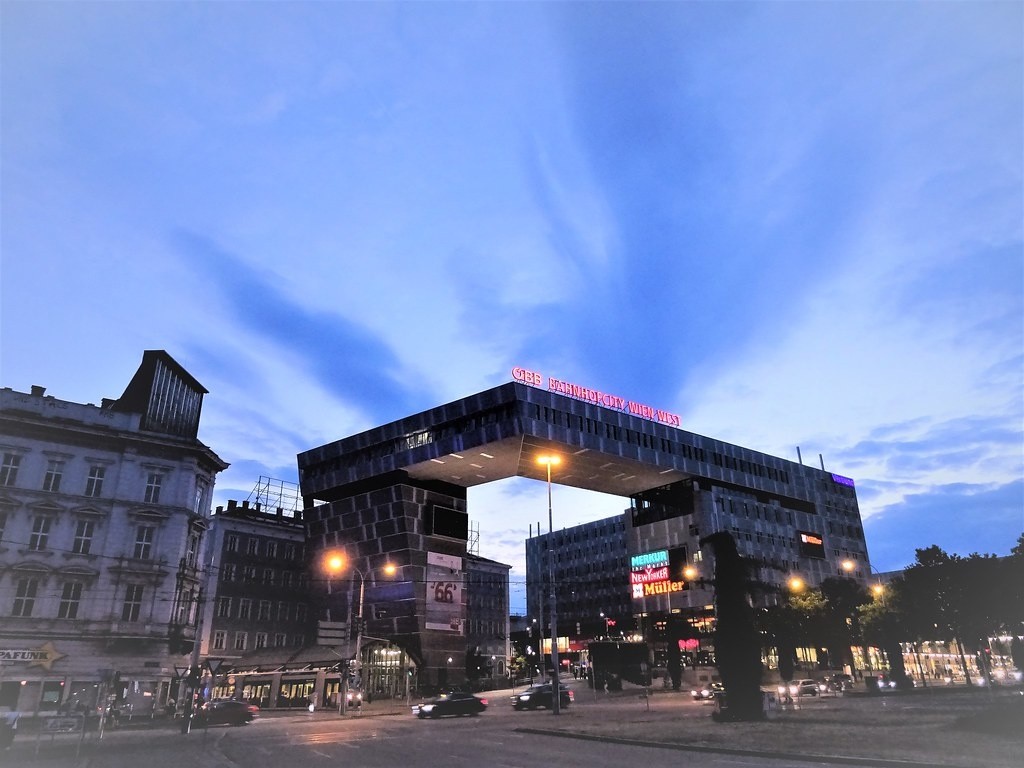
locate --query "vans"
[326,692,342,711]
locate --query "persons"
[603,680,609,694]
[109,697,133,728]
[2,706,21,750]
[168,695,177,717]
[573,667,578,679]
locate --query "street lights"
[352,632,390,712]
[324,551,397,717]
[181,555,261,734]
[682,567,698,605]
[535,456,562,716]
[842,560,882,609]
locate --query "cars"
[411,692,488,719]
[691,682,723,700]
[189,701,259,726]
[348,692,361,706]
[202,698,245,706]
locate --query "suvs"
[511,684,574,711]
[779,679,817,697]
[820,674,855,691]
